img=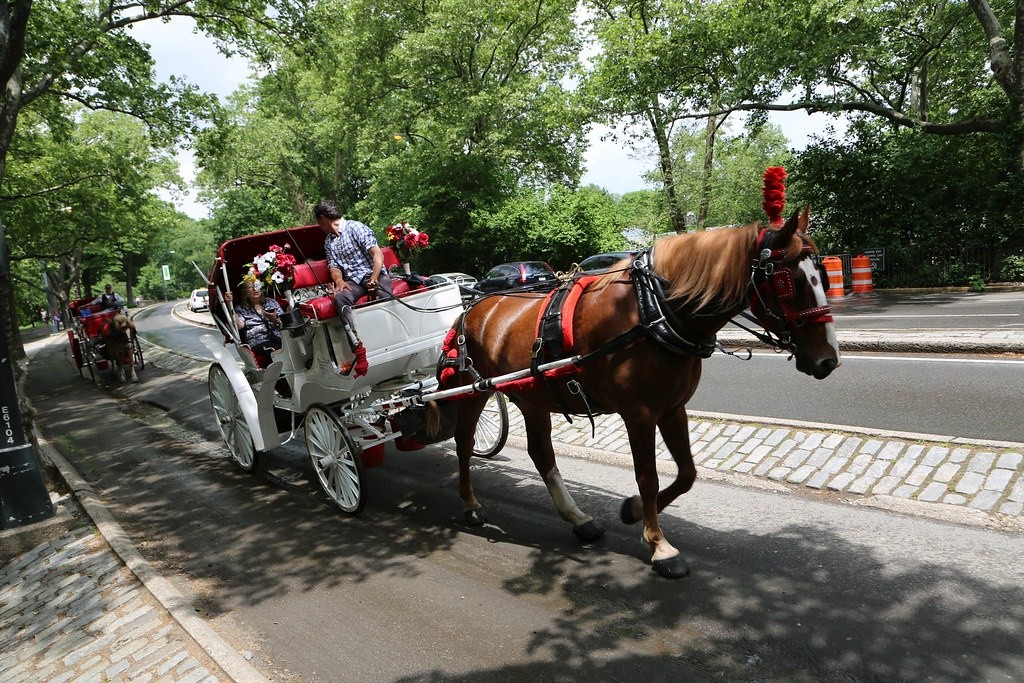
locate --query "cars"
[567,251,640,284]
[429,272,480,304]
[189,288,210,314]
[474,260,563,301]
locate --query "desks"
[295,247,402,291]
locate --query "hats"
[105,284,112,289]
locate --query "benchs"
[292,246,472,371]
[79,304,120,339]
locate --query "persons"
[40,308,62,332]
[312,198,392,353]
[225,280,285,362]
[77,283,124,316]
[135,295,140,305]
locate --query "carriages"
[64,291,145,386]
[200,206,843,580]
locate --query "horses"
[449,202,842,579]
[101,315,138,383]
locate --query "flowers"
[385,218,430,263]
[238,243,300,304]
